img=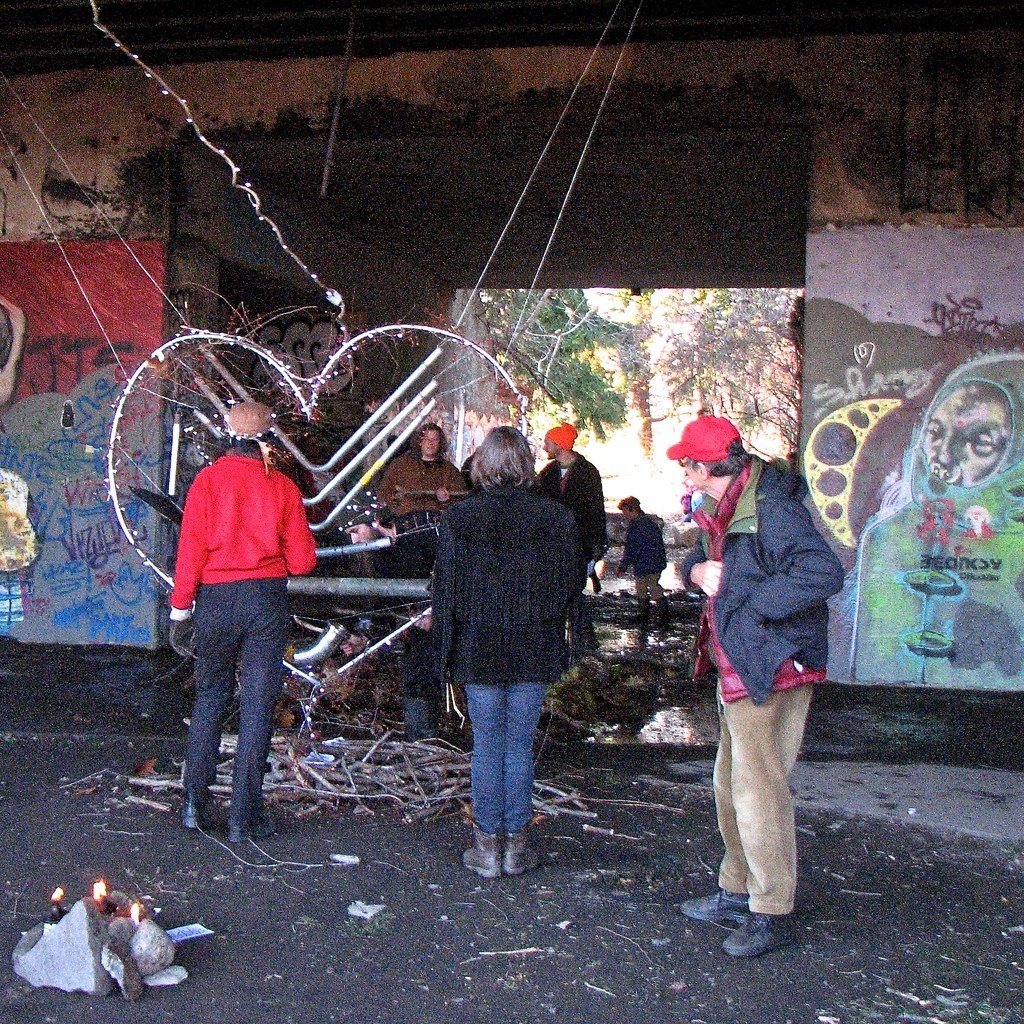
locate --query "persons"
[615,496,670,625]
[330,422,610,762]
[421,427,586,878]
[668,415,845,956]
[170,402,318,842]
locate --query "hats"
[668,414,742,463]
[546,422,578,452]
[226,402,273,438]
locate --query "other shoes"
[183,804,202,829]
[229,824,276,843]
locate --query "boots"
[462,829,502,879]
[504,830,538,876]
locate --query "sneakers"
[680,889,792,959]
